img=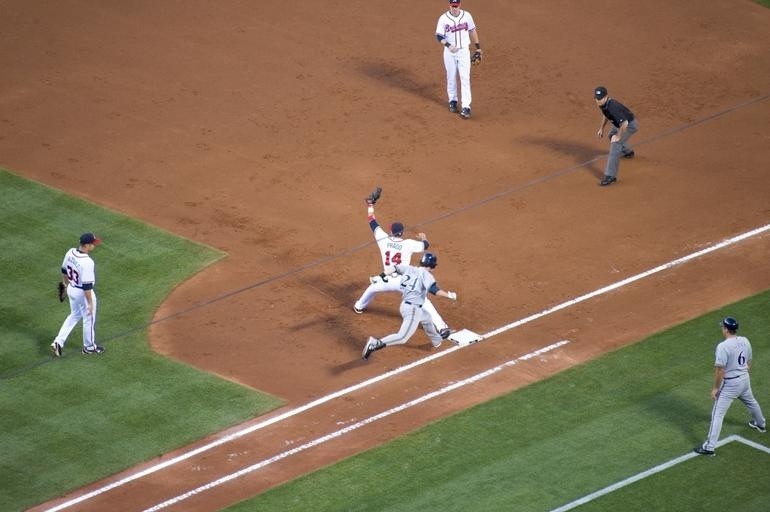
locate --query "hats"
[80,232,101,245]
[593,87,607,100]
[450,0,460,7]
[389,222,403,235]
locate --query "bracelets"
[445,42,451,48]
[368,208,375,213]
[476,43,481,50]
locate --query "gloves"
[370,276,382,285]
[447,291,456,301]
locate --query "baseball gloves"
[470,52,481,64]
[57,283,67,302]
[368,187,382,204]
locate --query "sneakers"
[51,341,62,358]
[449,101,458,113]
[749,421,766,432]
[361,336,382,360]
[82,343,105,354]
[601,176,617,185]
[461,107,470,118]
[693,447,715,457]
[353,304,363,314]
[440,327,449,339]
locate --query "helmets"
[421,253,436,263]
[718,317,738,330]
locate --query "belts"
[405,301,422,308]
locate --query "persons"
[434,0,482,118]
[361,251,450,360]
[693,318,767,457]
[350,187,458,339]
[48,232,105,357]
[594,87,638,187]
